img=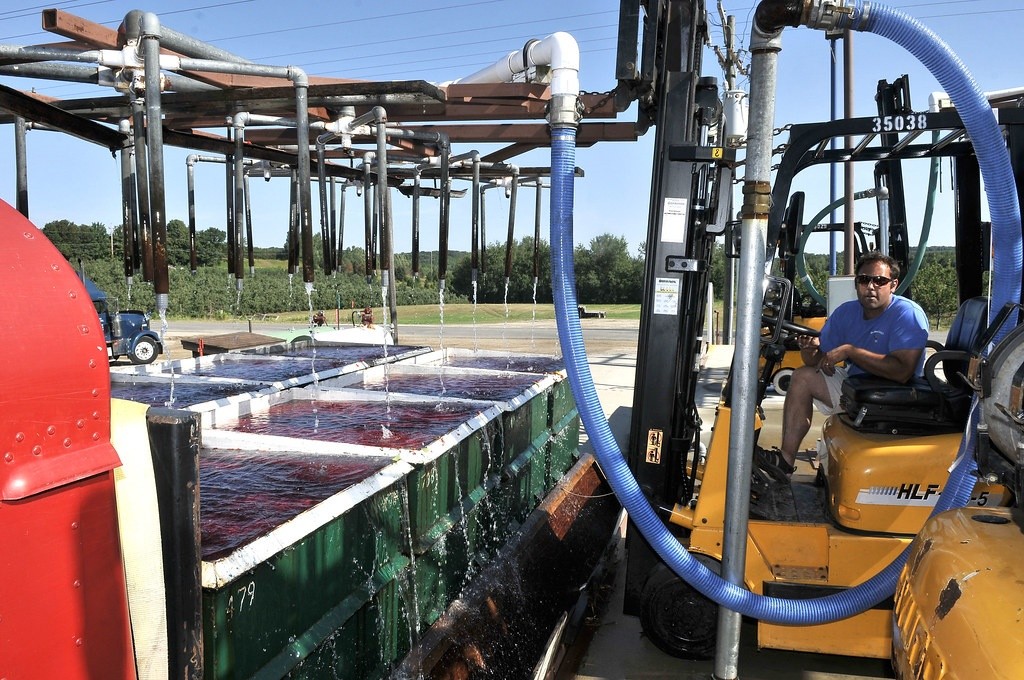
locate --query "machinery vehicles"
[748,187,910,398]
[636,82,1024,680]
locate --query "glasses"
[854,275,893,286]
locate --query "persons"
[754,252,929,484]
[359,307,374,328]
[310,311,326,328]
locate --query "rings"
[824,363,829,366]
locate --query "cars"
[73,257,164,364]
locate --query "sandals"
[752,444,797,485]
